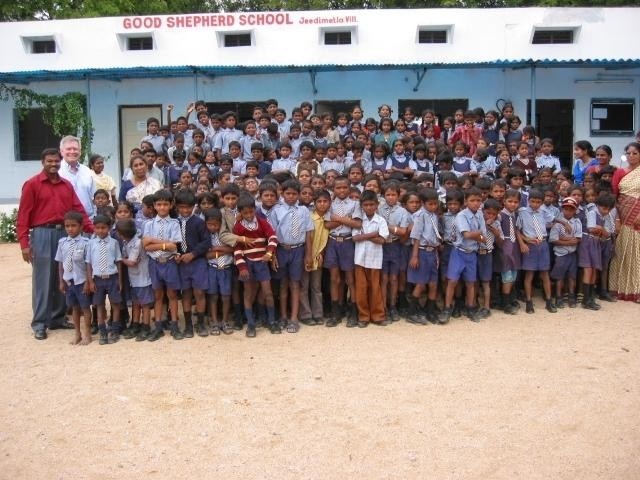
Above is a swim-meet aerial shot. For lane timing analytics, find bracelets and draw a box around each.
[266,252,272,258]
[394,225,397,235]
[215,250,219,259]
[162,242,165,251]
[242,235,246,243]
[615,219,620,221]
[479,234,484,242]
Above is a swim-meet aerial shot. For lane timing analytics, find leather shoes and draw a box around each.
[233,317,244,329]
[526,300,535,314]
[35,328,46,339]
[246,323,256,336]
[277,319,286,328]
[150,330,163,340]
[194,321,208,338]
[90,322,99,335]
[136,328,149,341]
[580,299,601,310]
[99,332,106,345]
[267,321,281,332]
[391,298,522,324]
[110,326,122,342]
[170,321,183,339]
[125,328,137,339]
[601,291,614,301]
[51,322,74,330]
[558,299,565,308]
[547,301,555,311]
[569,297,577,306]
[185,321,194,337]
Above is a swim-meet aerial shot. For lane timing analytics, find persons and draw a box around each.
[335,141,346,164]
[121,147,141,181]
[454,109,465,128]
[191,146,204,160]
[402,107,418,138]
[472,146,490,172]
[335,113,350,144]
[154,152,171,188]
[228,141,247,177]
[553,169,574,193]
[440,187,463,318]
[474,108,485,129]
[323,168,339,200]
[412,144,434,183]
[370,167,384,188]
[412,133,427,146]
[506,116,523,142]
[187,123,196,131]
[111,200,136,333]
[140,117,165,155]
[499,121,509,139]
[313,143,330,175]
[203,208,235,335]
[598,168,614,183]
[460,174,475,196]
[489,179,507,211]
[414,170,434,192]
[244,160,263,186]
[496,162,513,191]
[101,206,117,234]
[274,109,291,144]
[298,170,311,187]
[349,121,362,140]
[178,117,195,148]
[548,197,583,308]
[399,191,423,319]
[583,187,597,204]
[512,141,537,187]
[85,214,123,345]
[170,121,179,145]
[343,133,350,158]
[386,140,414,184]
[609,142,640,304]
[272,168,295,187]
[321,112,339,146]
[192,193,218,221]
[535,138,561,175]
[264,145,277,166]
[437,171,458,210]
[167,104,174,125]
[127,149,166,188]
[16,148,95,341]
[256,122,281,151]
[140,195,157,238]
[173,189,211,338]
[363,175,386,213]
[295,141,321,176]
[497,190,523,314]
[472,177,491,205]
[287,124,303,158]
[437,184,487,325]
[348,105,365,127]
[323,175,362,328]
[364,136,374,152]
[533,165,557,191]
[473,137,493,165]
[595,182,618,303]
[540,189,560,299]
[377,105,392,119]
[578,193,615,310]
[232,196,278,338]
[423,125,436,144]
[204,151,223,179]
[250,142,272,178]
[310,114,321,138]
[434,152,462,190]
[450,110,483,157]
[140,141,153,153]
[452,140,477,178]
[311,124,331,150]
[352,190,390,328]
[189,128,211,156]
[440,116,455,145]
[347,131,371,161]
[500,102,514,123]
[377,179,407,323]
[187,153,203,183]
[348,186,362,203]
[477,199,504,321]
[58,135,96,224]
[271,141,295,176]
[297,182,314,212]
[216,170,231,188]
[344,136,356,158]
[197,112,215,142]
[585,144,618,194]
[185,100,208,128]
[557,180,574,201]
[399,181,417,206]
[232,177,245,193]
[265,99,278,124]
[196,164,213,191]
[214,111,242,160]
[288,102,312,122]
[484,110,505,154]
[257,115,271,134]
[218,154,238,183]
[157,125,171,149]
[493,149,512,170]
[402,137,414,159]
[116,219,154,342]
[369,142,387,179]
[88,154,119,211]
[256,183,277,232]
[166,133,189,165]
[407,188,444,326]
[91,190,116,224]
[267,180,313,333]
[253,107,264,132]
[300,120,314,144]
[54,209,92,346]
[298,189,329,325]
[219,180,256,331]
[573,140,599,188]
[259,180,281,204]
[321,144,345,175]
[345,142,369,175]
[174,169,194,194]
[347,164,365,195]
[142,189,184,342]
[418,109,440,140]
[240,120,256,161]
[394,119,406,140]
[292,107,303,131]
[310,175,325,194]
[581,172,598,192]
[198,176,210,190]
[523,125,540,156]
[375,119,398,149]
[210,187,224,207]
[195,183,209,195]
[363,118,380,136]
[244,177,261,202]
[569,185,585,232]
[494,140,508,157]
[425,141,438,168]
[165,148,190,190]
[517,188,572,314]
[507,141,520,161]
[207,112,225,150]
[504,166,529,209]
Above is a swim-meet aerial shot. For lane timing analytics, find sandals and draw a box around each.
[222,321,232,334]
[288,321,299,332]
[210,323,219,336]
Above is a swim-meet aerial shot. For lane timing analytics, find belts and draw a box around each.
[328,235,352,242]
[419,247,437,251]
[36,223,65,229]
[93,274,118,280]
[209,264,231,270]
[152,257,177,263]
[280,244,305,249]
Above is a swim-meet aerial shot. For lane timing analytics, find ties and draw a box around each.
[153,220,165,258]
[214,232,224,268]
[432,216,442,241]
[509,216,516,242]
[181,218,187,253]
[67,240,75,271]
[486,229,494,250]
[561,226,570,241]
[450,216,457,242]
[99,240,108,271]
[532,211,542,241]
[385,207,390,226]
[336,199,344,230]
[292,208,298,239]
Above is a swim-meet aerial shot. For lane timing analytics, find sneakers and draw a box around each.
[326,317,342,328]
[316,318,324,325]
[302,318,314,326]
[346,317,356,327]
[375,321,388,326]
[357,322,368,327]
